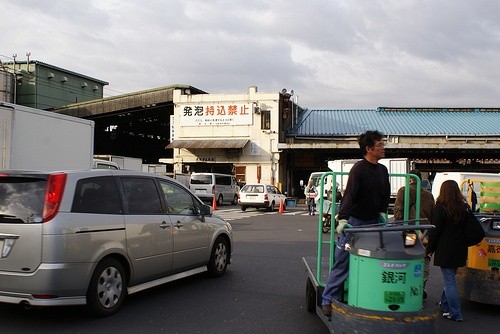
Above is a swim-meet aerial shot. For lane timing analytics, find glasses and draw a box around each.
[370,144,385,149]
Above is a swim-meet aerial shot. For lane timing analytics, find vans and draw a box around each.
[189,172,240,205]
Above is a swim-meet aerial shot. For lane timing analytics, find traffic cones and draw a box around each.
[210,197,218,210]
[278,198,285,213]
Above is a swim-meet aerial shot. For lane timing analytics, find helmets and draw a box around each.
[330,181,339,189]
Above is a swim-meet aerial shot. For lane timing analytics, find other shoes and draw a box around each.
[309,212,315,216]
[322,304,331,316]
[443,312,455,319]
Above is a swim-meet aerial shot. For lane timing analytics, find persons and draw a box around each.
[321,130,391,315]
[304,180,318,216]
[426,180,486,319]
[392,170,436,301]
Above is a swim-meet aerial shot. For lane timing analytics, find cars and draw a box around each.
[237,184,287,212]
[0,169,235,319]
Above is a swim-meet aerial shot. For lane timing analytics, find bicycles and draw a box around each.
[322,212,337,232]
[307,193,316,216]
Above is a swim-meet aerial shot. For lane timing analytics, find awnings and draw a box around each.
[165,139,249,149]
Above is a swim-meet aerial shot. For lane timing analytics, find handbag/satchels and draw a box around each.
[464,204,485,247]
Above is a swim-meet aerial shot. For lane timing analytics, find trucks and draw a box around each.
[93,155,142,172]
[300,158,415,212]
[430,171,500,209]
[0,101,119,171]
[142,164,166,176]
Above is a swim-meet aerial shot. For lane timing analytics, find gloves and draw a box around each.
[336,219,352,237]
